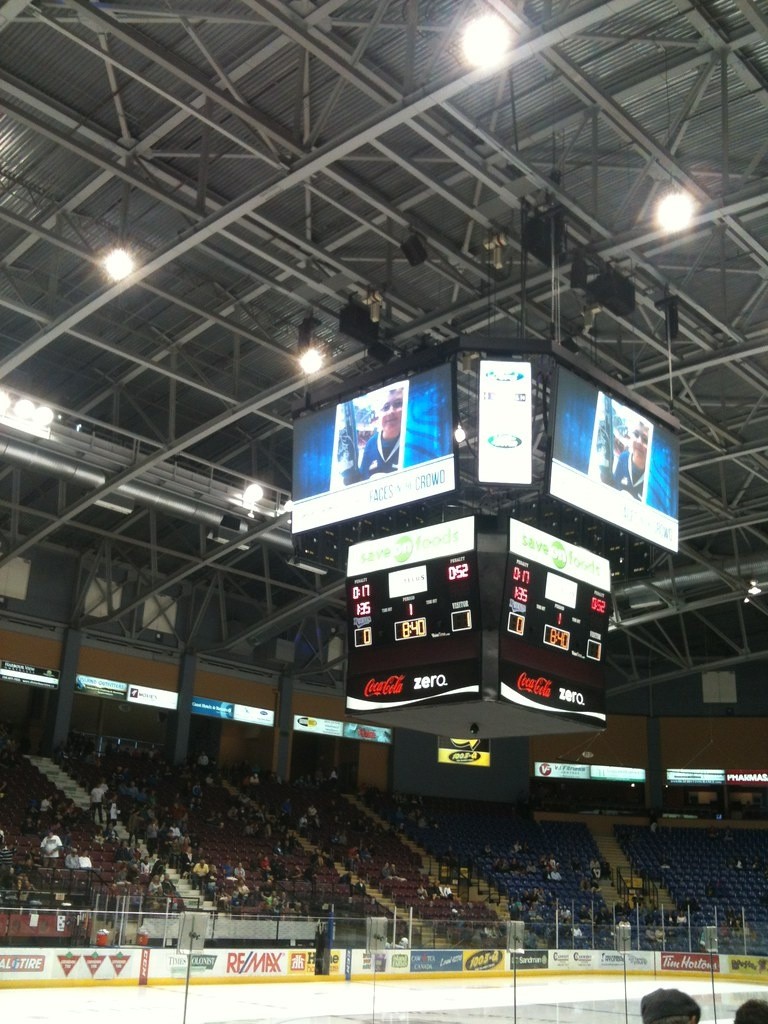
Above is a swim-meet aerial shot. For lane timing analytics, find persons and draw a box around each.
[734,999,768,1024]
[612,418,651,503]
[0,745,767,957]
[361,385,404,479]
[640,988,701,1024]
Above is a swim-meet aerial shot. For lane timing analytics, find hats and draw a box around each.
[641,988,700,1024]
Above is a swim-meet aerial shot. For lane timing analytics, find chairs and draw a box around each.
[0,749,768,955]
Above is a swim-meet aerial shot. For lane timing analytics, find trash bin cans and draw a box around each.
[97,929,110,946]
[136,926,149,946]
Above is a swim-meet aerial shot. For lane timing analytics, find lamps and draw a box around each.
[295,318,324,374]
[400,235,429,268]
